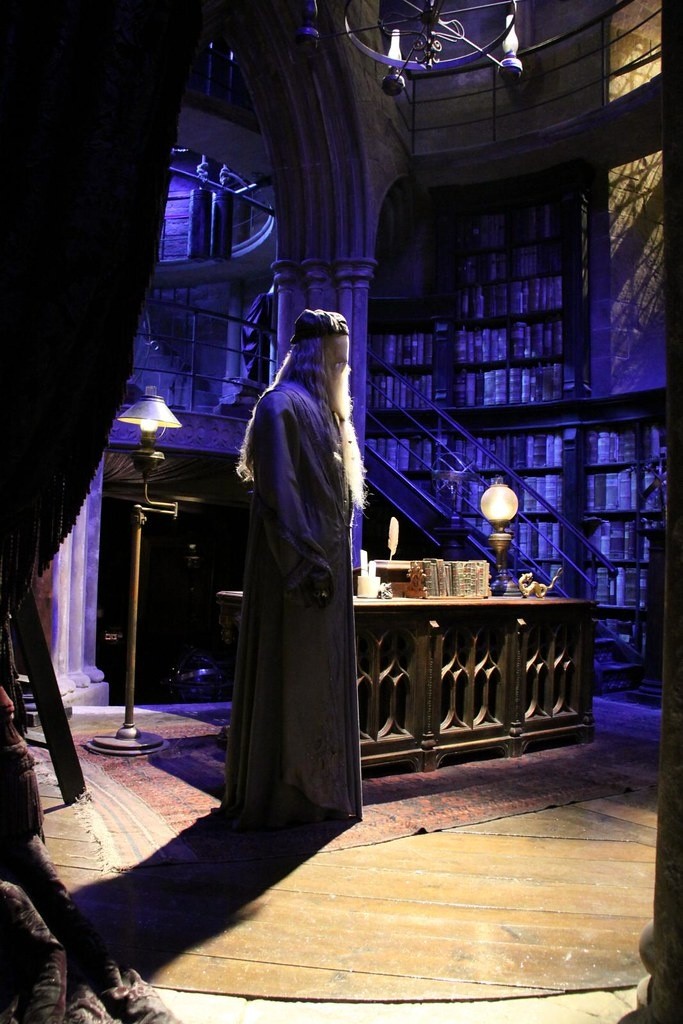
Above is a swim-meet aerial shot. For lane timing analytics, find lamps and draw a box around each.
[481,483,523,597]
[297,1,524,94]
[83,392,182,755]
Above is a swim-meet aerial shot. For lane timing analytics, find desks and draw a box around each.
[214,591,600,774]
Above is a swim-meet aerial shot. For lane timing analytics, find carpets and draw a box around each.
[26,696,669,872]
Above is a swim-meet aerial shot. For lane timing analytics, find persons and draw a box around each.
[212,310,368,829]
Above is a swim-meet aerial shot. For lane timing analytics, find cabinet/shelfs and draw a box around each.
[368,211,666,680]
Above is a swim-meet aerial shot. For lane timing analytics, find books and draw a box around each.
[534,562,648,653]
[365,198,668,558]
[411,558,490,597]
